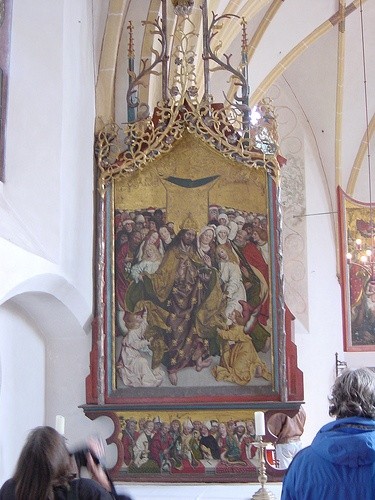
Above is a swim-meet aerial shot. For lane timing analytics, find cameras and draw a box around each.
[70,447,100,465]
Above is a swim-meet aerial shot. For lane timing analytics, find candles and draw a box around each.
[254,411,266,436]
[55,415,66,438]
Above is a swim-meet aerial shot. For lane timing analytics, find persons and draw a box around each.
[0,426,133,500]
[280,368,375,499]
